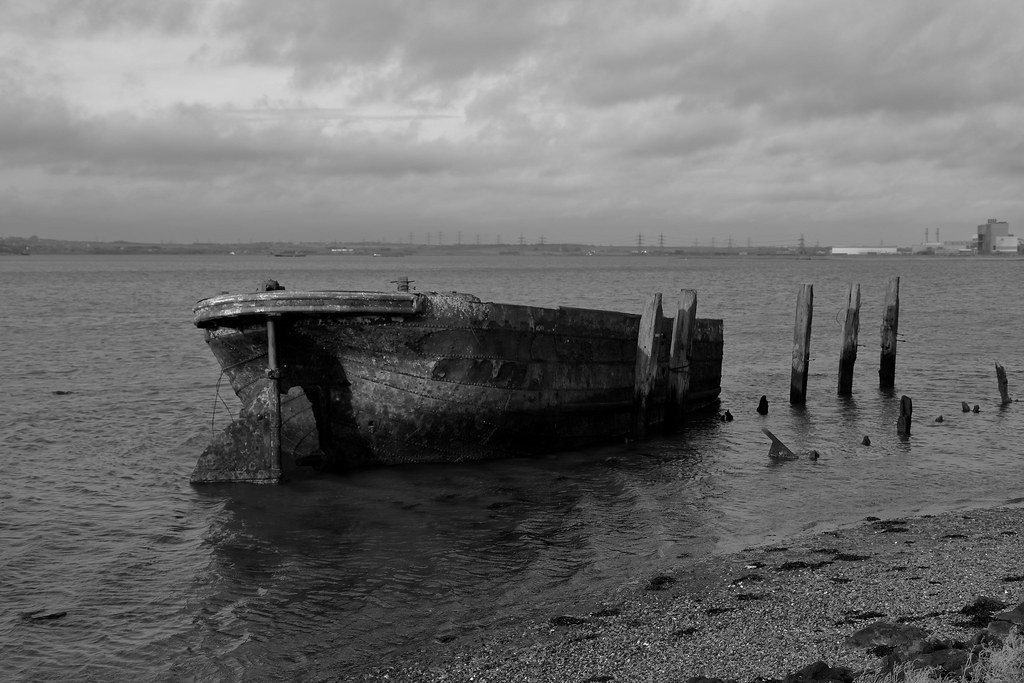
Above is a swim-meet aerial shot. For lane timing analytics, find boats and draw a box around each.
[189,275,724,489]
